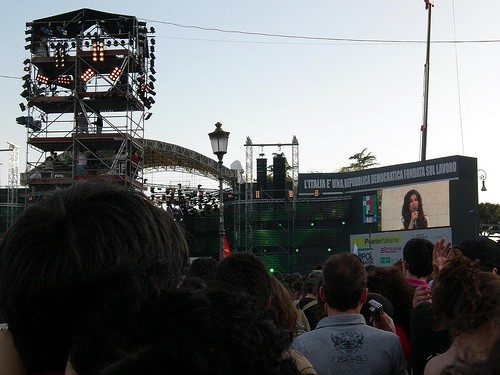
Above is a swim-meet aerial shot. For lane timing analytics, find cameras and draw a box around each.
[369,300,384,324]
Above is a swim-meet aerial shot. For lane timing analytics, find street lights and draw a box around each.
[208,121,230,262]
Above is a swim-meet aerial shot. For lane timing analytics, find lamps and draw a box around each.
[478,169,487,191]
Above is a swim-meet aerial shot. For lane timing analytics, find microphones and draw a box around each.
[414,208,418,227]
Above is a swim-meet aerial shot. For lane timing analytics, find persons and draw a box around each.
[50,149,58,162]
[63,238,500,375]
[95,111,103,133]
[77,149,87,178]
[86,149,98,176]
[132,151,139,179]
[0,177,189,375]
[400,190,428,230]
[78,110,88,133]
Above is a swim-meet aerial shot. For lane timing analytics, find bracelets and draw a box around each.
[300,366,314,373]
